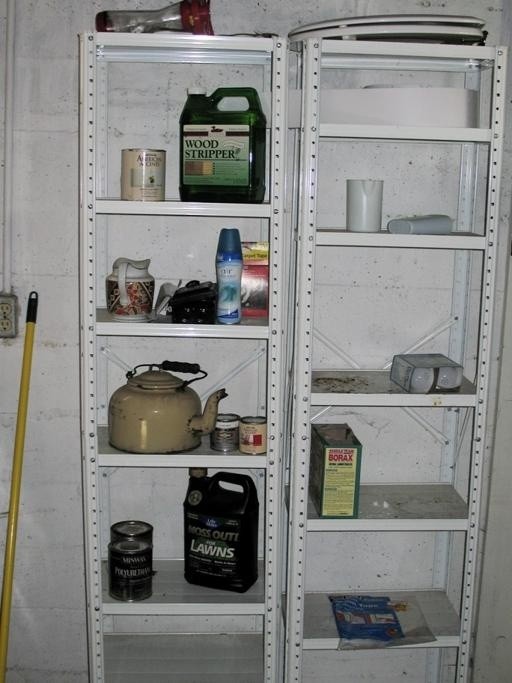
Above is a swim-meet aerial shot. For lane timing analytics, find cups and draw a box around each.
[345,179,384,232]
[212,412,268,455]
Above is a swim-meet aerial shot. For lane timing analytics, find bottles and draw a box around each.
[216,227,244,325]
[109,520,154,602]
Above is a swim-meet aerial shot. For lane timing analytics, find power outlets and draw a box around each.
[1,295,17,338]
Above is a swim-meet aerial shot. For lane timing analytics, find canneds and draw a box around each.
[121,147,166,201]
[110,520,154,543]
[107,543,154,603]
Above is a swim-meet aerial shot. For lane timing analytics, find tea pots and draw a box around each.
[107,360,228,454]
[105,256,155,322]
